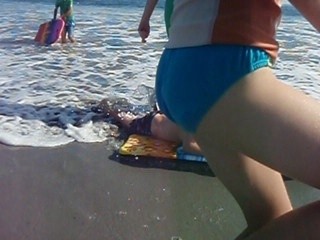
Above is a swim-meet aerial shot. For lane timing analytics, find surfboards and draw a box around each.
[34,19,65,45]
[119,134,209,163]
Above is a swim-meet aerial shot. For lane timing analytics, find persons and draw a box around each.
[138,0,320,240]
[54,0,76,43]
[96,100,204,156]
